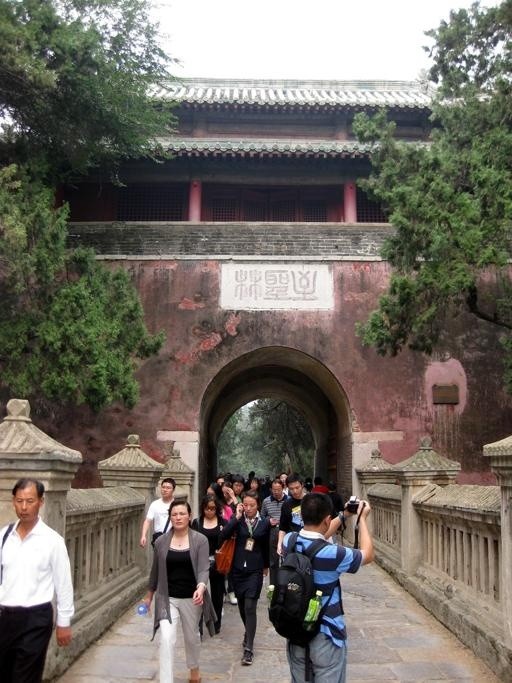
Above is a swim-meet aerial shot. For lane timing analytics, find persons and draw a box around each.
[218,492,271,665]
[140,478,176,615]
[282,493,375,683]
[143,500,218,683]
[192,471,344,644]
[0,478,75,683]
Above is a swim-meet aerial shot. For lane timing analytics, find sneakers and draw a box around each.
[215,627,220,633]
[241,632,255,665]
[226,591,238,604]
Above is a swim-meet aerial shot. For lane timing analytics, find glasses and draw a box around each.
[206,506,216,511]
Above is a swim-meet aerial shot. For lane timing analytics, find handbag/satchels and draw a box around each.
[151,532,163,548]
[214,531,237,575]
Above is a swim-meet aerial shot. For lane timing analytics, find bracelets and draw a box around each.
[338,511,347,522]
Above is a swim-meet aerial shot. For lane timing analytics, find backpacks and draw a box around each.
[268,531,339,648]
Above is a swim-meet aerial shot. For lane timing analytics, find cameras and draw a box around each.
[346,494,364,513]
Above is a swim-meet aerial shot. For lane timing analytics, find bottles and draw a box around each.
[137,605,151,621]
[304,589,324,621]
[266,585,276,610]
